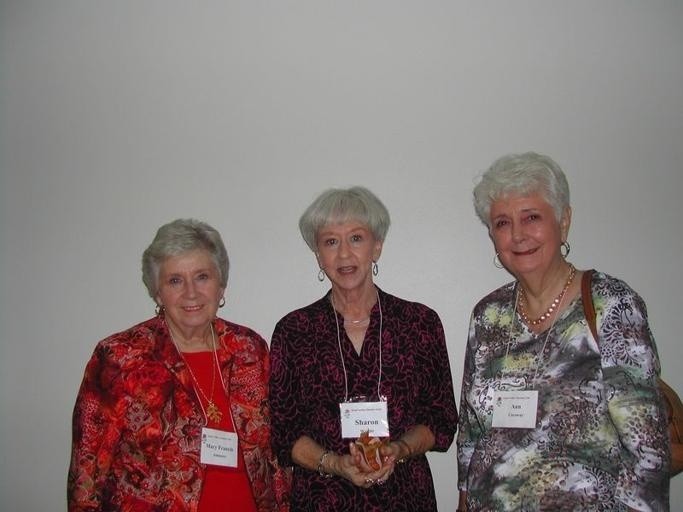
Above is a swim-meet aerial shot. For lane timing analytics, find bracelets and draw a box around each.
[316,453,332,480]
[391,443,408,466]
[399,438,412,457]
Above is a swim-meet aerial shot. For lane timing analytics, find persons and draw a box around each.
[451,151,672,512]
[265,185,458,512]
[67,214,293,512]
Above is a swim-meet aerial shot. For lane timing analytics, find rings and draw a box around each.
[366,477,375,486]
[384,454,394,463]
[378,479,384,487]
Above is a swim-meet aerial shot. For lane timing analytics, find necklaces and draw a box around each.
[166,326,223,425]
[343,317,371,326]
[515,265,574,325]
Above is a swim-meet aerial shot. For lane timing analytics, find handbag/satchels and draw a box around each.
[578,267,683,479]
